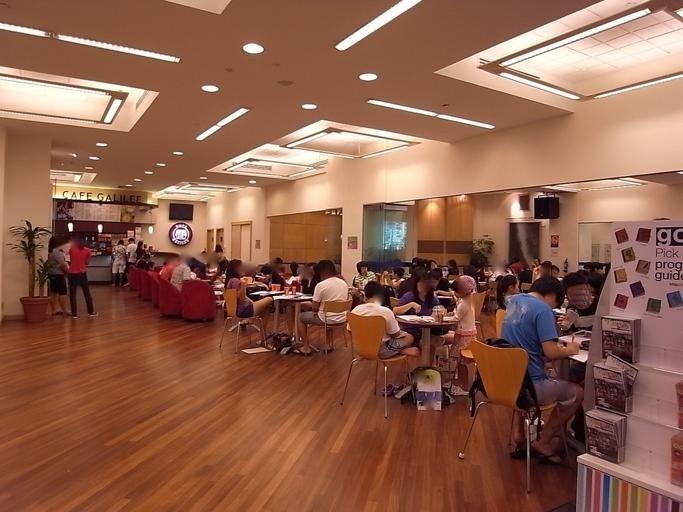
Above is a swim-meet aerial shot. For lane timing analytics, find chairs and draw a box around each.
[495,308,507,340]
[218,288,266,354]
[306,301,352,362]
[341,312,416,420]
[457,340,569,495]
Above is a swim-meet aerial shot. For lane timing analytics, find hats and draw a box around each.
[457,275,477,293]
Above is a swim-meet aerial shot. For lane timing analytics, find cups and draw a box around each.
[561,310,579,331]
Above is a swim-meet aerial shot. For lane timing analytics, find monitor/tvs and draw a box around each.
[169,203,194,220]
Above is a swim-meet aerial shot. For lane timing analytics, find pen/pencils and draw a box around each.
[571,335,575,343]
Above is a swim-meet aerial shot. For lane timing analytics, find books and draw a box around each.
[600,314,641,364]
[592,354,638,410]
[584,405,627,464]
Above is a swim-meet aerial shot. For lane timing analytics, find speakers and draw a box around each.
[533,196,559,219]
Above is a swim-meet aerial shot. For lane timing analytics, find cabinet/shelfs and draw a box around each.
[575,221,682,512]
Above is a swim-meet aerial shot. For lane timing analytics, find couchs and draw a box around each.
[157,277,181,318]
[181,279,214,322]
[135,269,140,295]
[151,274,159,308]
[140,270,152,303]
[128,265,135,292]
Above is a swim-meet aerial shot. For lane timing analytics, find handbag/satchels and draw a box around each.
[272,332,292,352]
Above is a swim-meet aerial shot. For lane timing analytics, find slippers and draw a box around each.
[381,383,398,395]
[510,445,531,458]
[448,386,469,396]
[530,450,562,465]
[393,383,407,395]
[443,378,455,389]
[292,348,312,355]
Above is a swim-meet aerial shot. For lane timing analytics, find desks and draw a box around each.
[253,289,314,355]
[557,330,590,439]
[394,314,460,405]
[76,247,127,285]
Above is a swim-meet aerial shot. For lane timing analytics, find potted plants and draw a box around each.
[5,219,58,322]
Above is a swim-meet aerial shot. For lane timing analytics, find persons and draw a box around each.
[261,256,286,285]
[500,275,584,463]
[192,263,218,282]
[529,261,560,291]
[583,264,591,272]
[48,237,69,316]
[136,240,146,256]
[446,275,477,395]
[67,232,98,318]
[393,271,440,371]
[137,254,150,269]
[287,262,302,293]
[380,271,388,286]
[301,262,321,295]
[591,262,604,275]
[497,275,518,309]
[352,261,376,298]
[398,266,425,298]
[392,268,406,288]
[123,238,137,287]
[511,257,524,274]
[448,259,459,276]
[532,259,540,268]
[556,273,598,335]
[111,240,127,286]
[346,281,420,357]
[433,268,450,296]
[447,265,489,311]
[224,259,273,345]
[292,259,348,356]
[425,259,437,272]
[170,264,190,290]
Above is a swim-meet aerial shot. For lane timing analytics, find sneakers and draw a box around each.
[72,314,80,319]
[88,311,98,317]
